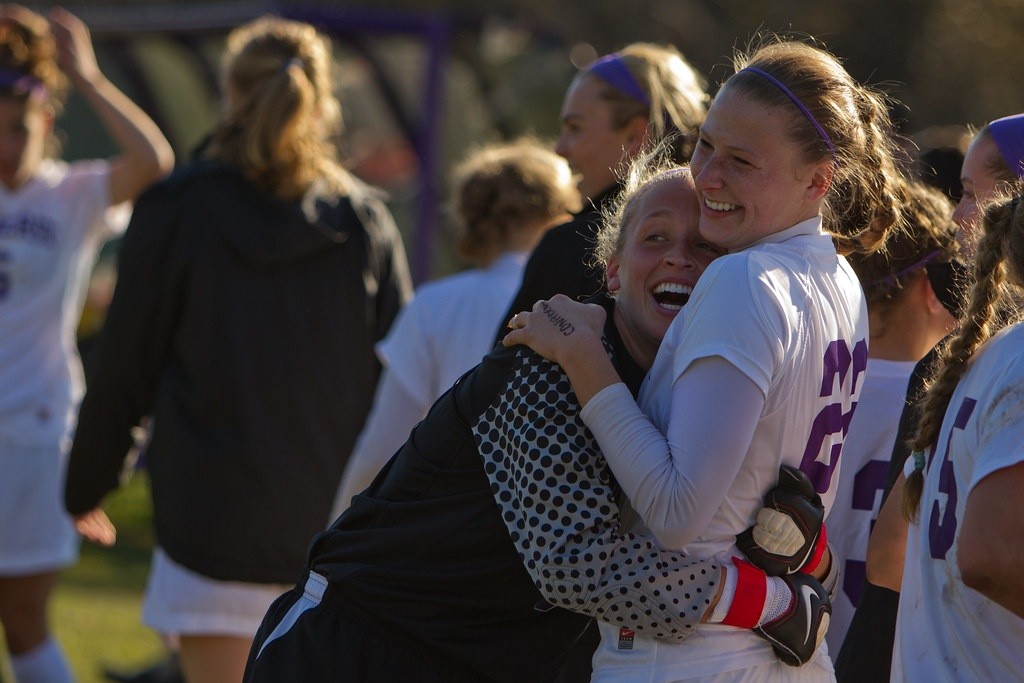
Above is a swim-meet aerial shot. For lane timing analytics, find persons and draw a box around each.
[0,6,173,682]
[494,40,707,344]
[503,40,894,683]
[243,167,836,683]
[65,14,415,683]
[828,113,1024,664]
[326,139,581,527]
[835,197,1024,683]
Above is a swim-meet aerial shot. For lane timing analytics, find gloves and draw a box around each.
[754,555,832,668]
[735,464,826,576]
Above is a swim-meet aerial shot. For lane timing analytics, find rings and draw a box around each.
[513,315,519,329]
